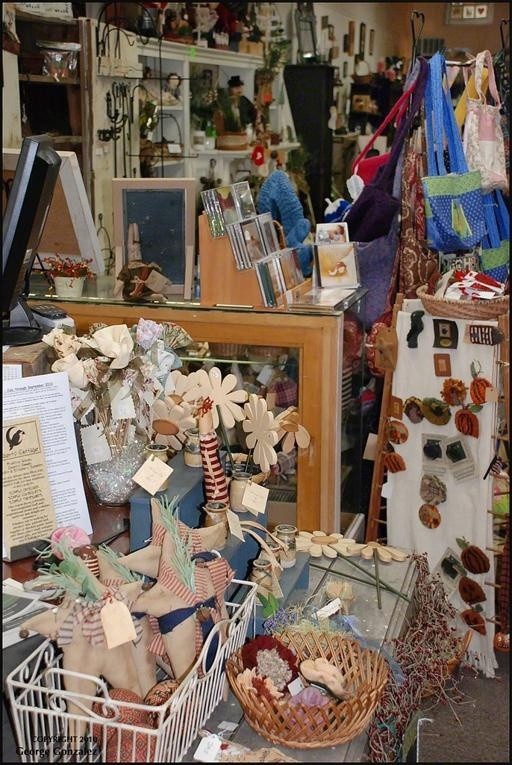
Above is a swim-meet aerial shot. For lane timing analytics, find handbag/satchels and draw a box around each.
[328,53,421,322]
[422,50,510,281]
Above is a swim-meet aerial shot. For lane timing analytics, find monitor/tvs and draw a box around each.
[2,135,61,345]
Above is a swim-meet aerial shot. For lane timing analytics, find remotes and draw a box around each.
[29,303,67,319]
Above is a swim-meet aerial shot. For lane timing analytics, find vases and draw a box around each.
[54,277,86,297]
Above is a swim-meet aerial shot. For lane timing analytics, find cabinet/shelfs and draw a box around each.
[284,62,337,224]
[27,287,369,534]
[15,14,144,278]
[138,36,301,209]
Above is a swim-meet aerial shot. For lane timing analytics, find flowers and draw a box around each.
[41,318,193,454]
[34,252,94,281]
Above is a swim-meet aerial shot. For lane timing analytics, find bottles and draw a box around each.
[205,501,229,542]
[251,559,271,607]
[184,427,205,469]
[276,524,297,569]
[145,444,168,493]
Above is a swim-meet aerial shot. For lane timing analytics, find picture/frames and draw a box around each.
[112,178,195,299]
[3,148,105,274]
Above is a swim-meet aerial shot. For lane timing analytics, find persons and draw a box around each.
[226,74,255,130]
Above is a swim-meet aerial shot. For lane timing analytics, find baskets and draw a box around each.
[224,628,392,752]
[415,277,509,322]
[6,575,259,761]
